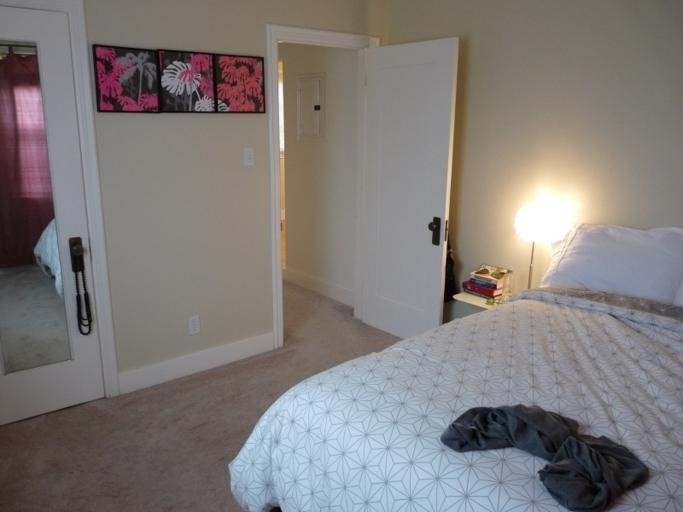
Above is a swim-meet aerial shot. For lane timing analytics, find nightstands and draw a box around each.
[450,294,511,322]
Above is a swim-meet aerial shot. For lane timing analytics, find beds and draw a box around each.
[227,253,683,511]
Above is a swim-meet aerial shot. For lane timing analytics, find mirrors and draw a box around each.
[0,39,73,376]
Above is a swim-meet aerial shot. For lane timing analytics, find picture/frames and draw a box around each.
[91,42,160,113]
[156,46,217,114]
[213,52,265,114]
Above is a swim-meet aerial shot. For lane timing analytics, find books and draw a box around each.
[461,264,514,300]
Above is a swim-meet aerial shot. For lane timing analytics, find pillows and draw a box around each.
[539,222,683,305]
